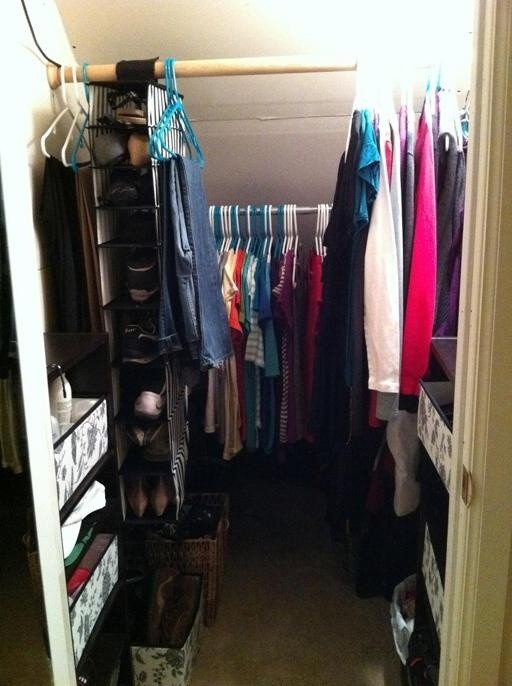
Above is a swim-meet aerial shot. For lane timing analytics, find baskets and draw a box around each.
[124,490,231,628]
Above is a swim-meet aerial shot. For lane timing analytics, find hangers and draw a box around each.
[148,58,206,169]
[42,63,90,172]
[344,54,472,164]
[206,203,330,290]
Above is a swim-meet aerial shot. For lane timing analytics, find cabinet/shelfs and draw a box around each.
[44,331,132,686]
[405,337,458,686]
[87,79,189,523]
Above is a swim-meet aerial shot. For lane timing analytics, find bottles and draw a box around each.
[50,363,71,425]
[50,416,60,442]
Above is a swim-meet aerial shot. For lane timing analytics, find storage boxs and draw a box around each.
[421,519,449,648]
[51,391,110,514]
[415,378,456,492]
[130,572,206,686]
[65,531,120,670]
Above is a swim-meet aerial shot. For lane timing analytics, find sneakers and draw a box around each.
[162,573,200,648]
[145,565,179,646]
[93,90,175,518]
